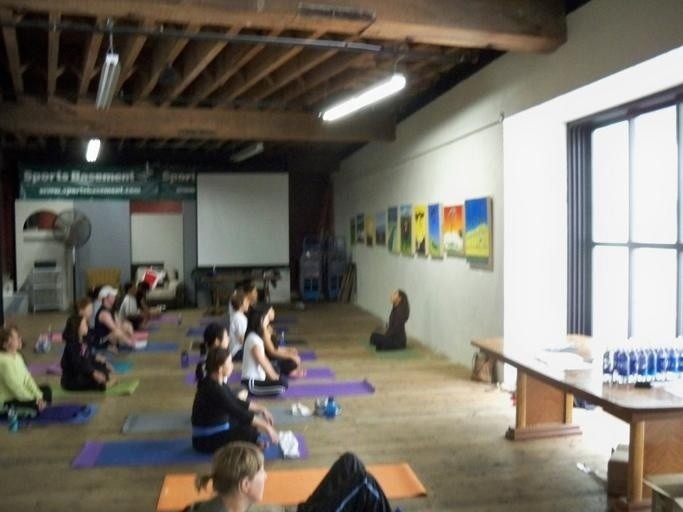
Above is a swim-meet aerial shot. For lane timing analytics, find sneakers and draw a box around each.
[32,331,62,353]
[290,398,343,420]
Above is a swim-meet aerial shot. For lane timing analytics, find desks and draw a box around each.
[472,333,683,511]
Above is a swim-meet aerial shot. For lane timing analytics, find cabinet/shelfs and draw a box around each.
[32,263,64,314]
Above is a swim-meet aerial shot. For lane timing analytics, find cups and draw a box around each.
[176,312,184,327]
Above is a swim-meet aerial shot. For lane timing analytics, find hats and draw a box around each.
[98,286,118,299]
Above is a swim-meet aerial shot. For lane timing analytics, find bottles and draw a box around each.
[35,331,46,352]
[279,330,287,347]
[7,405,19,431]
[180,351,189,368]
[326,396,338,419]
[603,346,683,384]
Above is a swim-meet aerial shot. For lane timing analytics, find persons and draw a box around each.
[370,290,409,351]
[60,281,161,391]
[191,280,392,511]
[0,326,52,421]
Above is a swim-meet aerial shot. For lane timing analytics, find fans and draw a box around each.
[53,209,92,319]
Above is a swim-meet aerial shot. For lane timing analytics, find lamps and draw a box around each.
[94,18,123,112]
[317,52,407,123]
